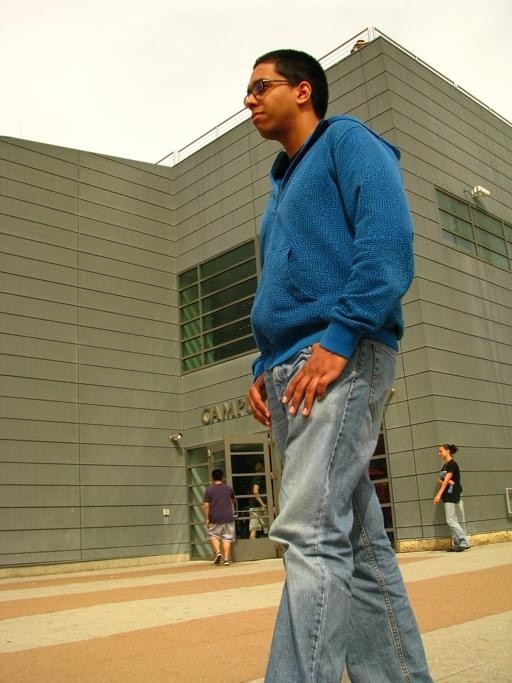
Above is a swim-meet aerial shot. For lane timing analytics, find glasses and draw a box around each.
[244,79,314,108]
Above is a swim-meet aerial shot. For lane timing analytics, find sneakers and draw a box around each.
[223,561,229,565]
[215,552,222,564]
[448,547,471,551]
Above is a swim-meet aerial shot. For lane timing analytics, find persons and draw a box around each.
[245,48,434,683]
[247,462,270,539]
[203,469,238,565]
[432,444,471,552]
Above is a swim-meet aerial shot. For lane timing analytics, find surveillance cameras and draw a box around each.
[471,185,491,199]
